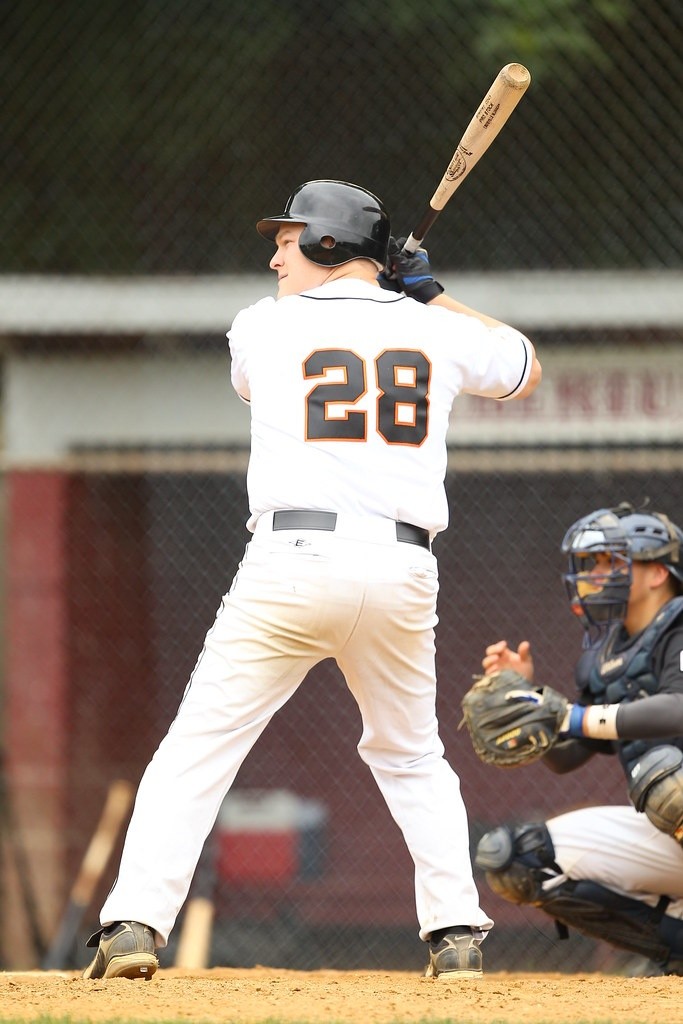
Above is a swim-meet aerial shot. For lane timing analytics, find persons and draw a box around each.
[475,503,683,976]
[84,181,543,981]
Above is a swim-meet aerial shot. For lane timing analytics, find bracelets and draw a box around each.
[569,703,585,738]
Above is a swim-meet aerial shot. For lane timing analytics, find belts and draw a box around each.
[273,510,430,552]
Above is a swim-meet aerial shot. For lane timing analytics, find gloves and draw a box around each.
[376,236,445,304]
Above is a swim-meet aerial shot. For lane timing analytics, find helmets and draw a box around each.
[562,513,683,563]
[257,180,391,275]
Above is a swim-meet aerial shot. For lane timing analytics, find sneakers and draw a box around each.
[82,922,159,981]
[426,934,483,979]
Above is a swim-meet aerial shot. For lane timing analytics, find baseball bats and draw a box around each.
[391,63,531,274]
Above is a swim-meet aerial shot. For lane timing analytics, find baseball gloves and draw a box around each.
[455,669,568,770]
